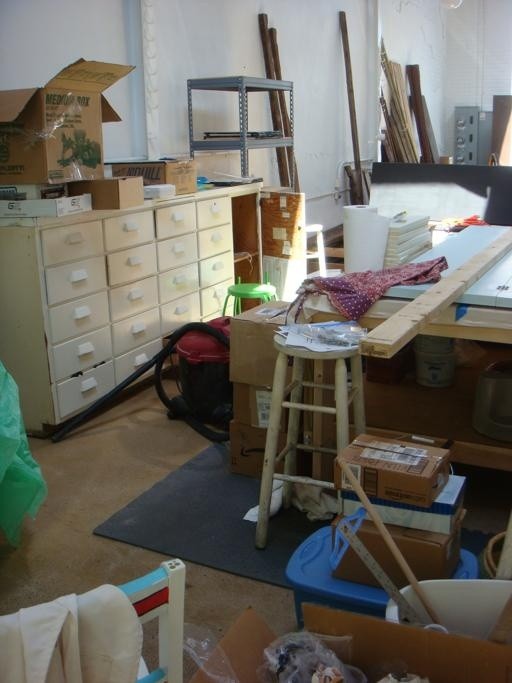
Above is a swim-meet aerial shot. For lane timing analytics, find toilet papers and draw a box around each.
[343,205,390,274]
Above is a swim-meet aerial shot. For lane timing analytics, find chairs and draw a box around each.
[0,557,186,683]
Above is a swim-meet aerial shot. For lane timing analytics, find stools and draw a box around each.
[222,284,278,316]
[307,223,328,274]
[255,324,366,548]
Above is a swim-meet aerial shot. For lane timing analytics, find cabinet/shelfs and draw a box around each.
[187,76,296,191]
[0,181,269,441]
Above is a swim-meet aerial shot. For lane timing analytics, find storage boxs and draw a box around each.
[112,160,198,194]
[0,59,137,187]
[68,175,146,210]
[236,383,287,433]
[330,515,464,591]
[189,600,512,683]
[337,476,468,536]
[287,520,480,632]
[333,436,455,509]
[229,300,309,389]
[227,420,288,476]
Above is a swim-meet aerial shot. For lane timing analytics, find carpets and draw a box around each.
[90,439,330,590]
